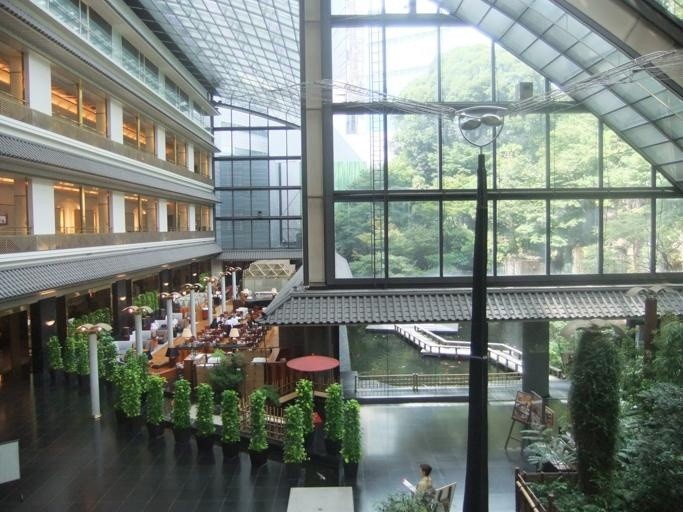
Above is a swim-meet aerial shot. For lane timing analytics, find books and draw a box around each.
[400,479,416,494]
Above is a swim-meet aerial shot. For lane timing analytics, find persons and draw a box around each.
[212,311,241,328]
[412,464,432,497]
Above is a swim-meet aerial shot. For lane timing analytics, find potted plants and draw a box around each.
[45,335,123,402]
[144,377,165,436]
[245,385,270,466]
[281,406,309,479]
[342,400,363,478]
[193,382,218,451]
[322,382,345,456]
[171,378,192,443]
[219,389,241,456]
[116,348,150,418]
[294,379,314,450]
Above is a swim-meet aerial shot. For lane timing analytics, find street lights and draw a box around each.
[219,267,243,314]
[122,306,154,355]
[77,322,113,420]
[203,276,218,325]
[159,291,180,356]
[182,283,203,337]
